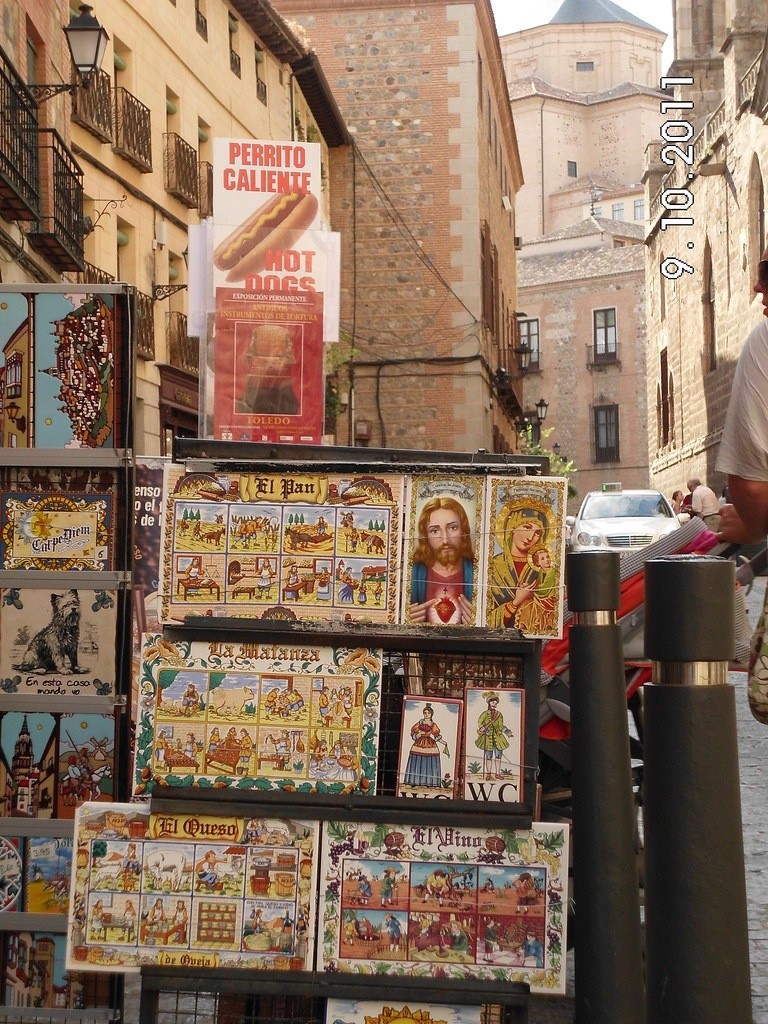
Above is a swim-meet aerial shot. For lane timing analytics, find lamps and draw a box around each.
[515,397,549,433]
[552,442,561,455]
[11,4,110,132]
[151,245,188,301]
[490,339,534,389]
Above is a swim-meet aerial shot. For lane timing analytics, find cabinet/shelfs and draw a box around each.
[139,437,550,1024]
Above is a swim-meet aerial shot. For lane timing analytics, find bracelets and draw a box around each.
[689,509,692,512]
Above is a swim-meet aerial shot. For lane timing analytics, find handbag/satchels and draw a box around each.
[747,582,768,725]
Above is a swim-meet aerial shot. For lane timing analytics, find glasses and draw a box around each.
[757,260,768,286]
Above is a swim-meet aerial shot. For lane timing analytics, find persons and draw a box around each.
[681,492,697,519]
[671,490,684,515]
[682,478,721,533]
[718,486,729,507]
[716,244,768,548]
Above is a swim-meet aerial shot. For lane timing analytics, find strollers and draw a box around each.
[497,517,768,1024]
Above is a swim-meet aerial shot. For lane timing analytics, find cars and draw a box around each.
[565,482,692,557]
